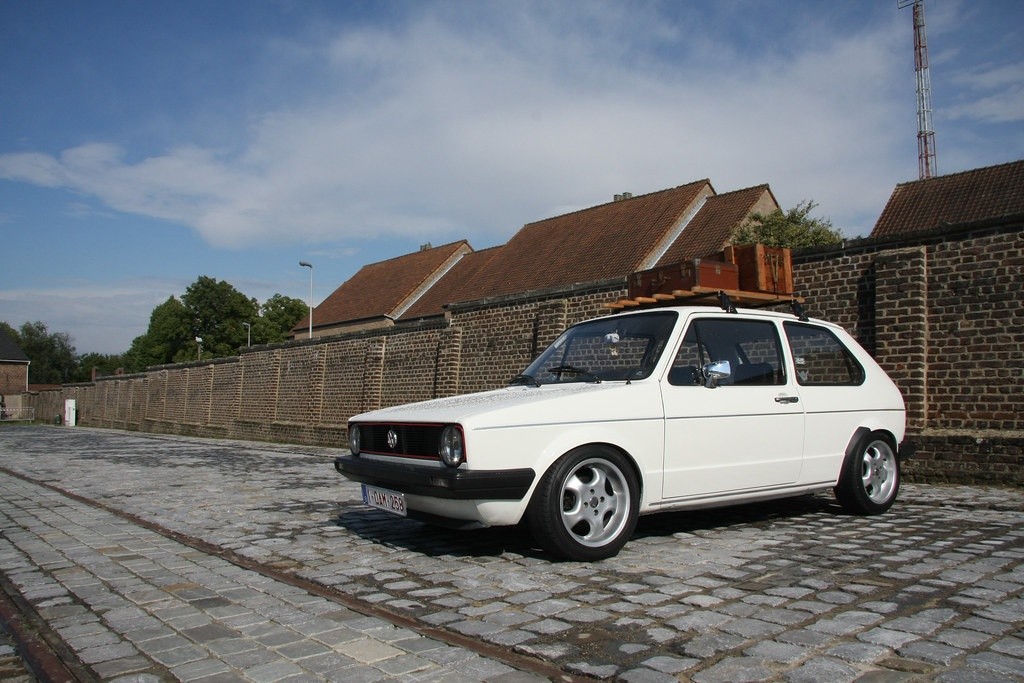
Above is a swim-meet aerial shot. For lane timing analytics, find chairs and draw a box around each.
[734,362,774,385]
[670,365,696,385]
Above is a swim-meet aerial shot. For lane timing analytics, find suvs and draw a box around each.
[334,292,908,563]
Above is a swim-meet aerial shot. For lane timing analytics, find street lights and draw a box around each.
[299,260,313,339]
[242,322,250,347]
